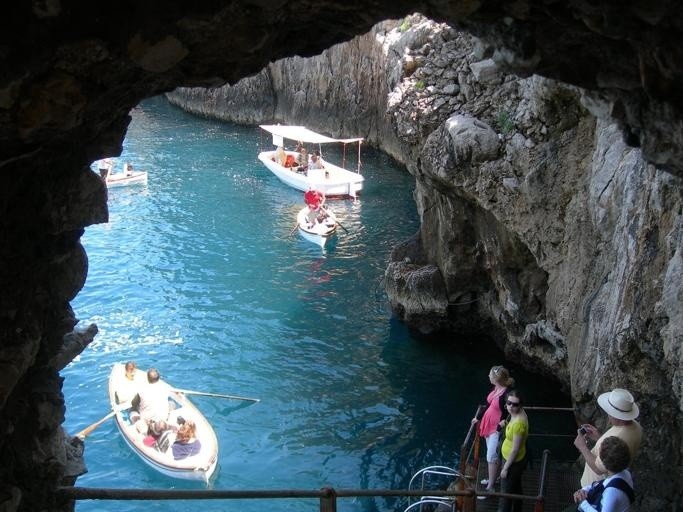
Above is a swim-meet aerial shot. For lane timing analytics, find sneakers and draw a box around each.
[477,487,495,500]
[481,476,501,485]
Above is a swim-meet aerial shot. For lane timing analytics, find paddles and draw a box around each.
[320,206,348,233]
[292,210,310,235]
[173,389,261,402]
[74,408,118,436]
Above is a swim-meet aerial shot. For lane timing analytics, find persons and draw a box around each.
[115,360,139,403]
[129,367,178,432]
[470,363,515,501]
[304,184,327,231]
[146,420,175,455]
[494,389,530,512]
[170,422,201,462]
[271,143,325,177]
[571,436,639,512]
[571,386,644,492]
[97,157,113,181]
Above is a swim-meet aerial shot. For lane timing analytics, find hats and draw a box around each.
[597,388,640,421]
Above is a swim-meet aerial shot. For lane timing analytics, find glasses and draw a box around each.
[506,400,521,408]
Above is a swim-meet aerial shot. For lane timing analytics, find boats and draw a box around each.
[109,363,219,483]
[297,206,337,247]
[258,125,365,197]
[106,171,148,189]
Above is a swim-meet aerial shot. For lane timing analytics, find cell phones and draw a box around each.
[580,427,588,435]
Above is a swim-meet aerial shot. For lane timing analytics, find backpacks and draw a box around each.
[577,477,636,512]
